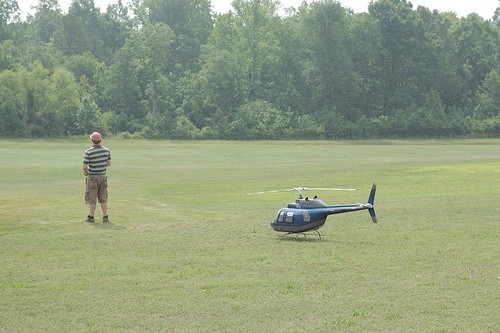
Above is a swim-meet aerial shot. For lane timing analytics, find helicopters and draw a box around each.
[246,182,378,243]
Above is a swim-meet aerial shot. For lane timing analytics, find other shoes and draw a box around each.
[103,215,109,222]
[84,215,95,222]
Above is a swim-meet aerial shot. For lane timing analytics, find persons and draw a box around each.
[82,131,111,221]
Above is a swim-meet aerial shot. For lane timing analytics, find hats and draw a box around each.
[90,132,102,140]
